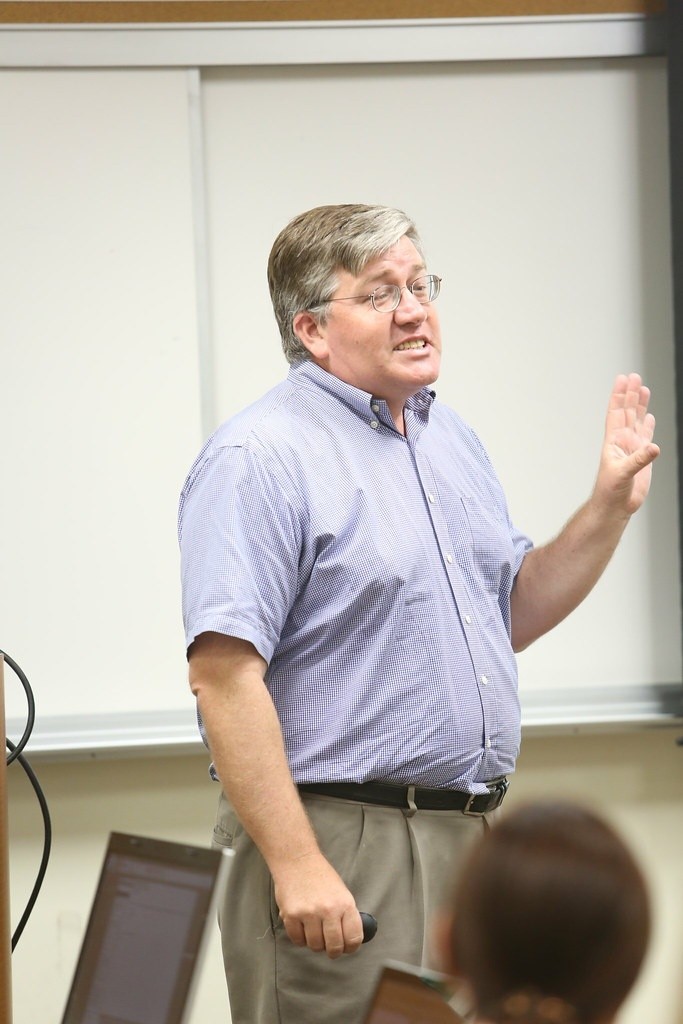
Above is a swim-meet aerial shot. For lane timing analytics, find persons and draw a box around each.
[178,204,660,1024]
[435,795,653,1024]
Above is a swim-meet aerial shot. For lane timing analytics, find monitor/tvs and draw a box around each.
[356,961,471,1024]
[58,829,236,1024]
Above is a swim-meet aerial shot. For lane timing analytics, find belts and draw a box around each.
[295,779,510,816]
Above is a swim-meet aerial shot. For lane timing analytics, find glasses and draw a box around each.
[313,274,443,313]
